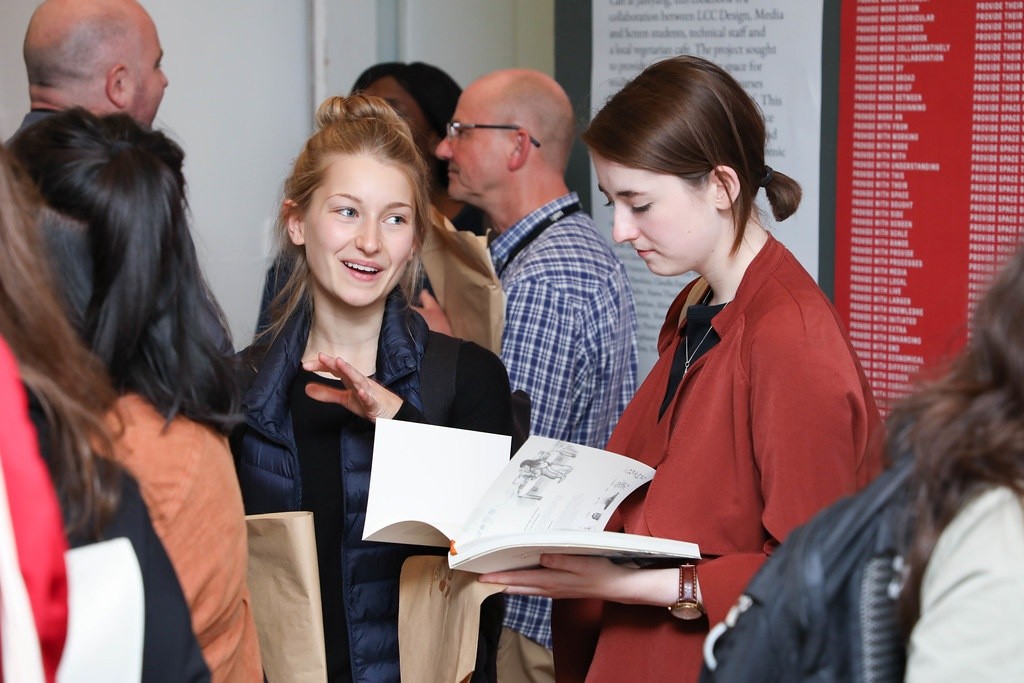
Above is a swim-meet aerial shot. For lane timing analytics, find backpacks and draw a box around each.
[699,449,1006,683]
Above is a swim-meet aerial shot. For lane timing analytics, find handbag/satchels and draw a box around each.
[240,511,328,683]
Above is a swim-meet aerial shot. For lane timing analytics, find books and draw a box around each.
[360,417,703,582]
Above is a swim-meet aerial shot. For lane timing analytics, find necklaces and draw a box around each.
[683,289,732,379]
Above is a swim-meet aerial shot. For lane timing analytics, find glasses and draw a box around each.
[446,121,541,148]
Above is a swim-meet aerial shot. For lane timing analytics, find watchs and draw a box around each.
[669,564,705,620]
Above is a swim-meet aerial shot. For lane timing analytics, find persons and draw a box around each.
[877,246,1024,683]
[477,55,879,683]
[229,92,515,683]
[253,61,483,360]
[0,106,272,683]
[17,0,168,137]
[404,69,640,683]
